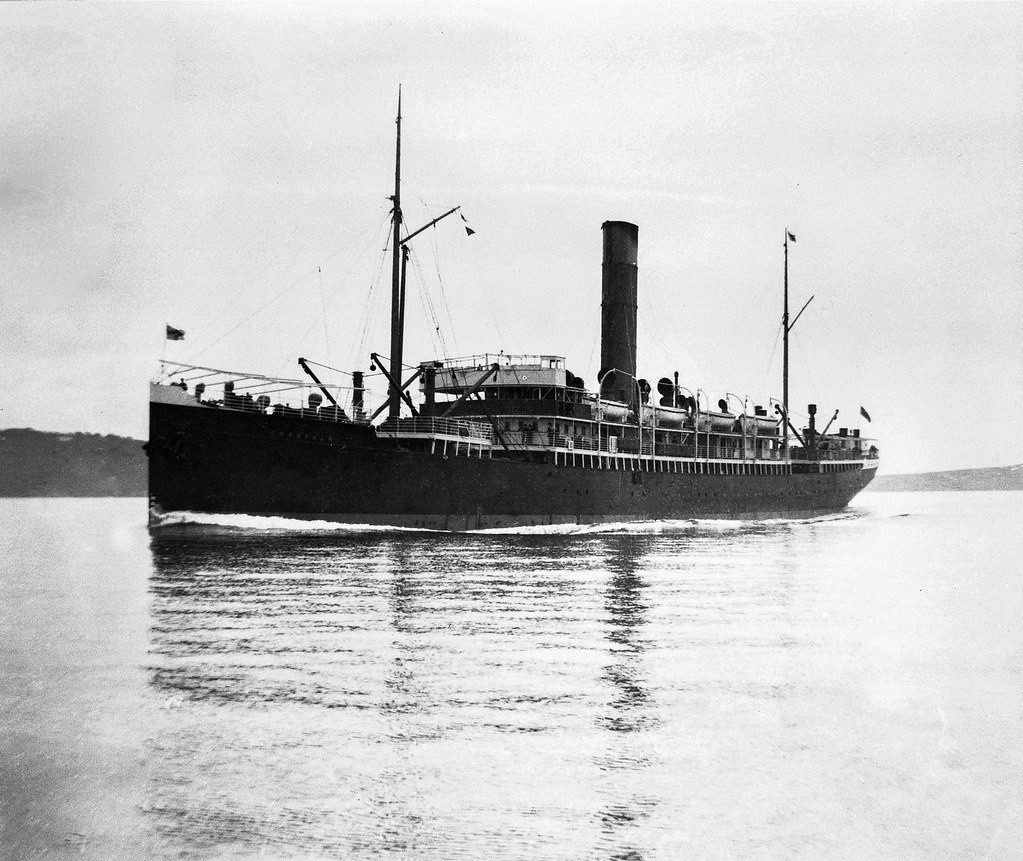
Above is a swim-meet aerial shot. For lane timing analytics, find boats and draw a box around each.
[142,83,880,545]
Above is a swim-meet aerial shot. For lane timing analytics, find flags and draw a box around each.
[789,233,796,241]
[861,406,871,422]
[166,325,185,340]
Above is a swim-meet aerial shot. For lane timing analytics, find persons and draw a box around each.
[180,378,188,391]
